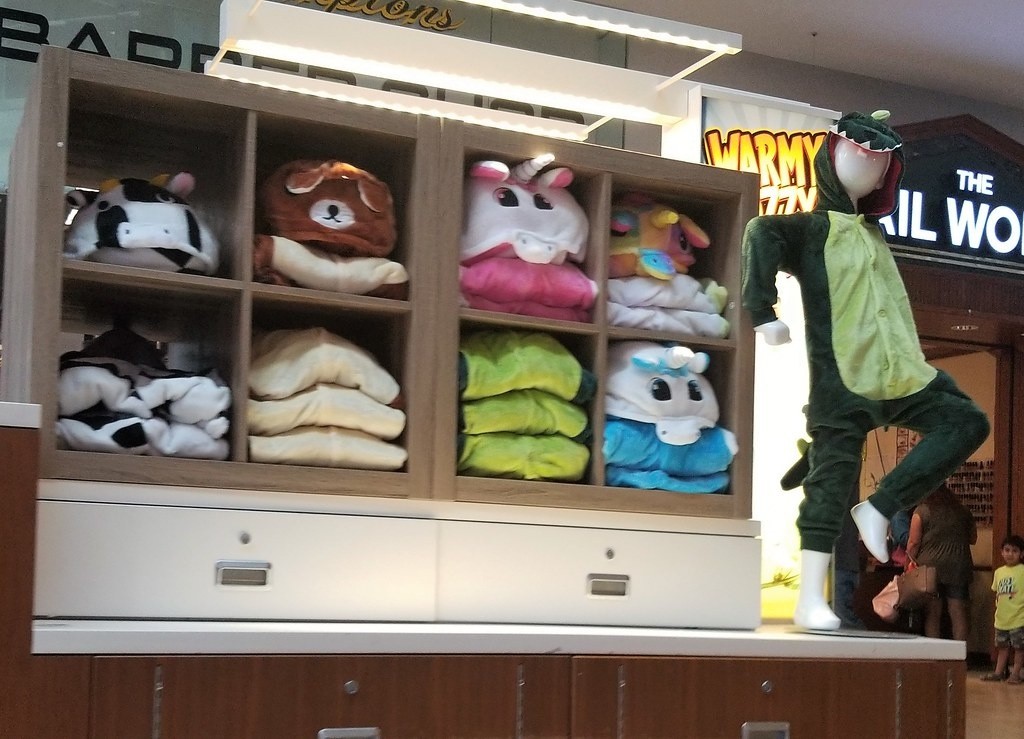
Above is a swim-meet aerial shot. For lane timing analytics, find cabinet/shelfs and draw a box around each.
[87,651,967,739]
[1,43,762,630]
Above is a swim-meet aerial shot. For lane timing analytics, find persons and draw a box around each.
[741,113,992,630]
[893,482,977,640]
[980,535,1024,684]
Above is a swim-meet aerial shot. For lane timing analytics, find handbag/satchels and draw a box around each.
[893,553,938,613]
[871,575,899,623]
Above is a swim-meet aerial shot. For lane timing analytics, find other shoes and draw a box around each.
[1006,675,1024,683]
[979,669,1010,681]
